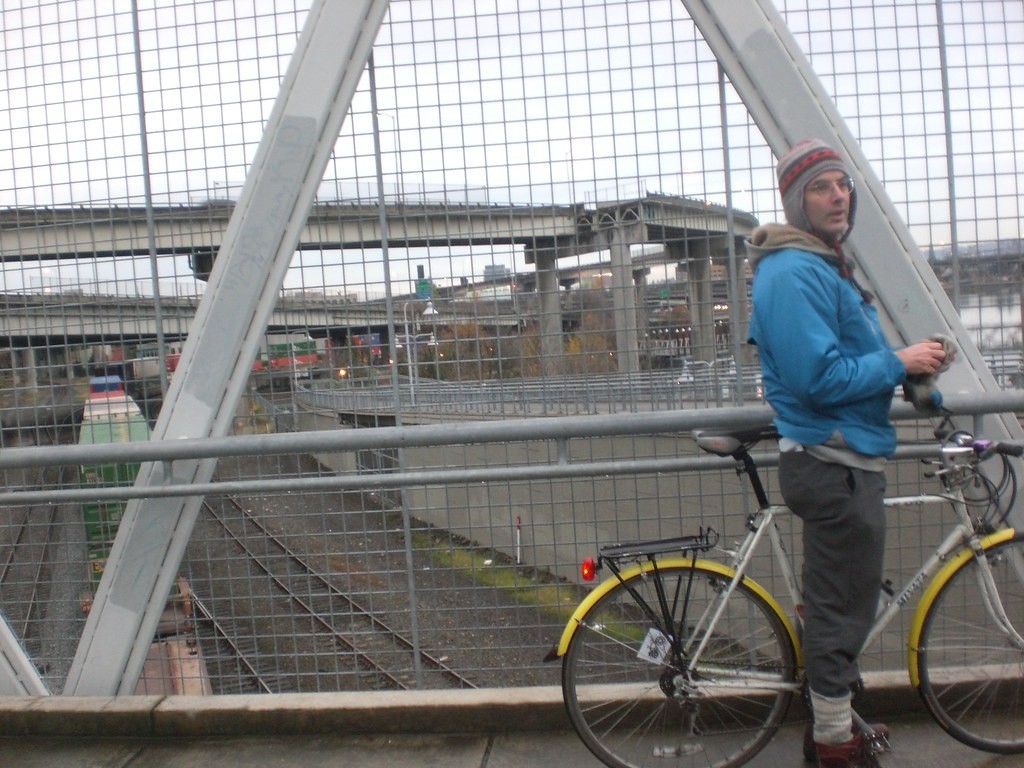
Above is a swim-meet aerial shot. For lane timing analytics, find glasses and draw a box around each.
[804,176,854,197]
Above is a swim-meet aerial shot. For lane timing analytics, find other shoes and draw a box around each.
[803,724,889,759]
[812,727,881,768]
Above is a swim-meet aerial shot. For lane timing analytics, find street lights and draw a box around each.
[290,331,314,394]
[676,355,737,398]
[403,293,437,407]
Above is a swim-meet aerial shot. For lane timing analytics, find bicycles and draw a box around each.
[542,424,1024,768]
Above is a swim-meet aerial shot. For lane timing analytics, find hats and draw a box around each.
[776,138,875,303]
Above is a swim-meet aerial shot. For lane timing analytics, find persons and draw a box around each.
[746,139,957,768]
